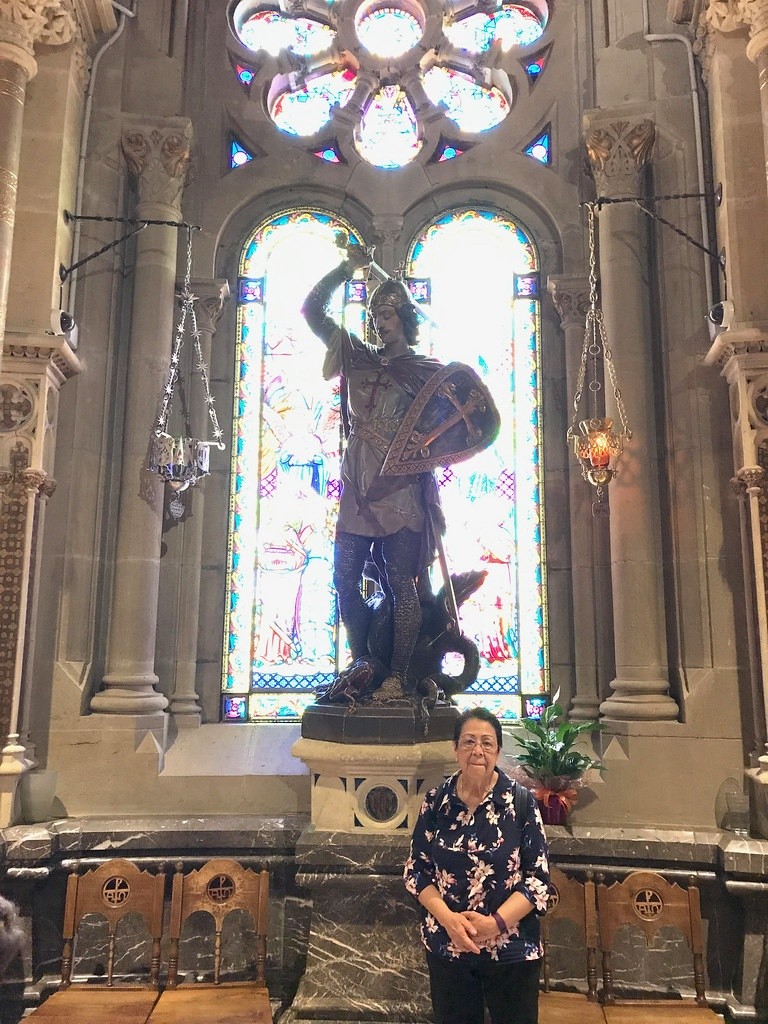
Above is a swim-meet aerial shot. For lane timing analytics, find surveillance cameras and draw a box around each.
[51,310,75,336]
[709,300,734,328]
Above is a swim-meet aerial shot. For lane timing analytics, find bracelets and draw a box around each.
[491,912,509,936]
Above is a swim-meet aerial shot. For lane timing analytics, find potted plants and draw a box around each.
[501,684,610,827]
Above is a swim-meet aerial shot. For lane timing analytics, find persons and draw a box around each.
[300,245,447,702]
[403,707,551,1024]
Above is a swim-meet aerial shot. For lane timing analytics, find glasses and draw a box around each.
[456,736,500,753]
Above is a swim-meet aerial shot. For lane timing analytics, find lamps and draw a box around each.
[566,182,727,517]
[62,208,226,518]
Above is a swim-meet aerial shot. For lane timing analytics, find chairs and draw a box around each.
[597,871,725,1024]
[538,866,607,1024]
[18,858,165,1024]
[146,859,273,1024]
